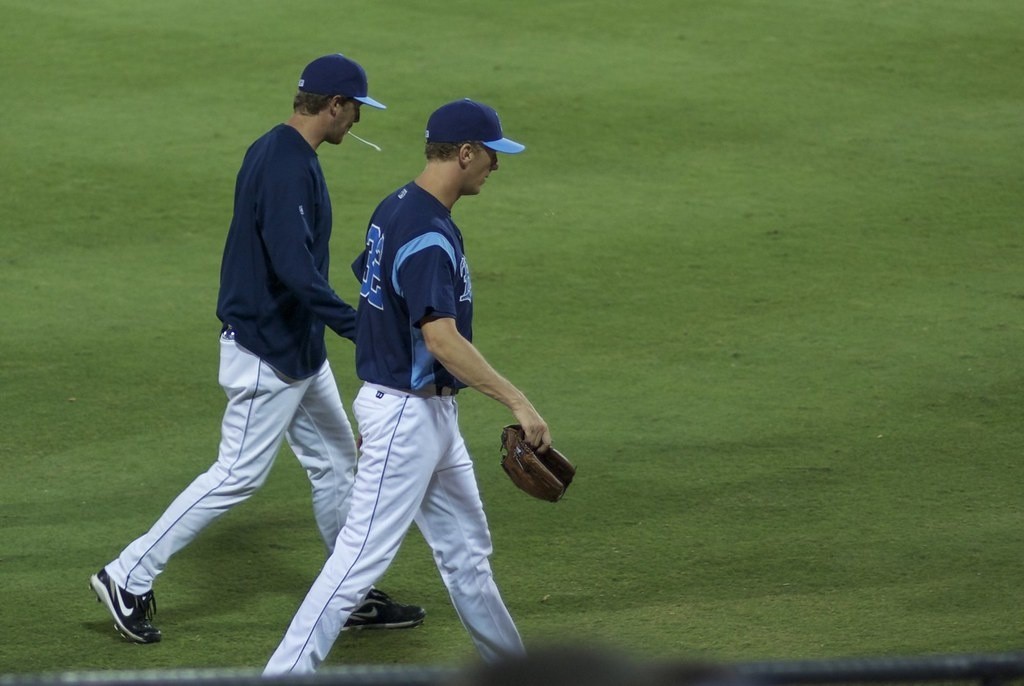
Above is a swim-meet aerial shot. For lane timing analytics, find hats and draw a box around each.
[425,98,525,154]
[297,53,387,110]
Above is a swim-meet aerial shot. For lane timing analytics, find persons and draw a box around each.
[260,97,553,676]
[88,54,426,644]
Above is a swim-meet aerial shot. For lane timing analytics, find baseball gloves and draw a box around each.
[498,423,579,503]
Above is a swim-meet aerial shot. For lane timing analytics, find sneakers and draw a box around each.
[88,570,162,645]
[340,588,425,631]
